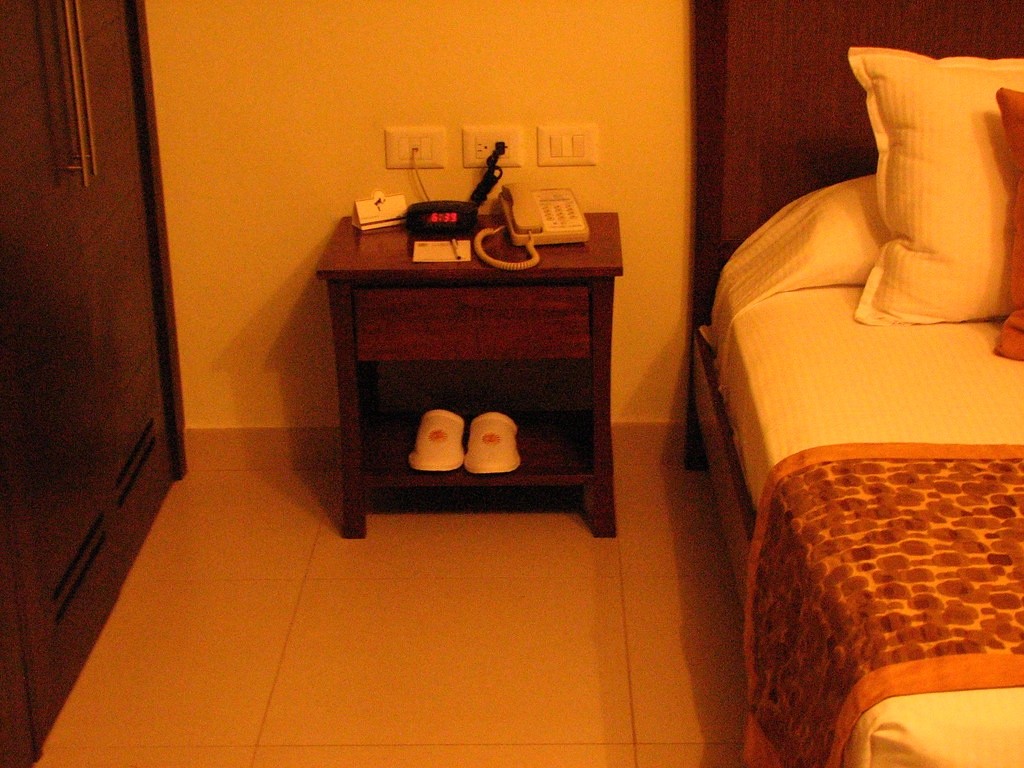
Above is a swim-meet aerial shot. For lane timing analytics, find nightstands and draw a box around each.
[315,209,624,539]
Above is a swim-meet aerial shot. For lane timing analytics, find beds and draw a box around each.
[685,0,1023,767]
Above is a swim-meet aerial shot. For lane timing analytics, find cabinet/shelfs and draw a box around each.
[0,0,190,768]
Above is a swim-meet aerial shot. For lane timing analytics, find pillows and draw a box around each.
[845,45,1023,322]
[699,167,881,396]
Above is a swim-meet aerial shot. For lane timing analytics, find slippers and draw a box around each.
[463,411,521,474]
[408,409,465,472]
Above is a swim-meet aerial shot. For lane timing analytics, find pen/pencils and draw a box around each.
[451,238,461,259]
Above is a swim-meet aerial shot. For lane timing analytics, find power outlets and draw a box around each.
[463,126,524,168]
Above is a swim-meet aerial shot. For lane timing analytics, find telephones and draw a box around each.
[499,183,590,246]
